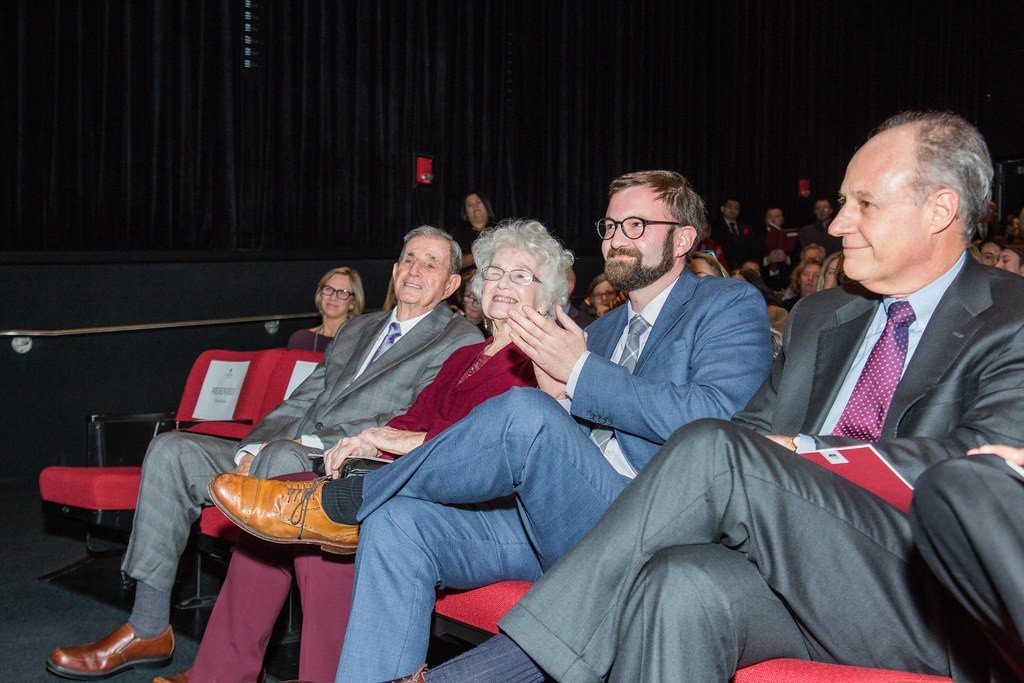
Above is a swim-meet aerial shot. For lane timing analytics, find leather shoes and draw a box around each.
[46,623,174,680]
[154,668,193,683]
[206,473,359,555]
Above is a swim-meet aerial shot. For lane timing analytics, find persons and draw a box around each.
[377,109,1024,683]
[909,443,1024,683]
[43,224,486,683]
[452,184,1024,379]
[205,170,772,683]
[189,220,574,683]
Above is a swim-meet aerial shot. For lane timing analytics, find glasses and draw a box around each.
[462,295,478,304]
[703,250,717,257]
[592,290,615,299]
[320,284,354,300]
[481,266,542,286]
[596,217,680,239]
[816,206,831,211]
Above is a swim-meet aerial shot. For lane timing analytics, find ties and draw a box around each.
[588,315,650,455]
[366,322,401,371]
[832,301,916,444]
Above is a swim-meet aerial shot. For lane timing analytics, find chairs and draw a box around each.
[37,349,957,683]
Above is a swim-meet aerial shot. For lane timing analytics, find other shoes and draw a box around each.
[385,664,428,683]
